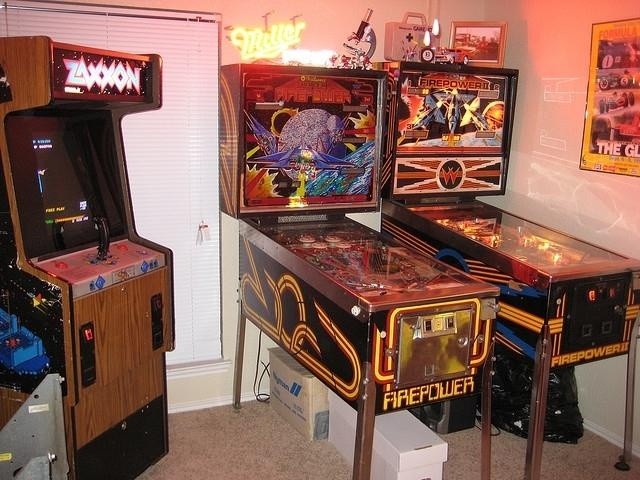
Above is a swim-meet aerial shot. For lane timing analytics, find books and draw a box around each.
[268,346,330,443]
[327,387,450,479]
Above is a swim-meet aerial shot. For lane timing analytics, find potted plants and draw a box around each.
[448,19,508,68]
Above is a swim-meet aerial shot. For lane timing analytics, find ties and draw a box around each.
[4,112,128,259]
